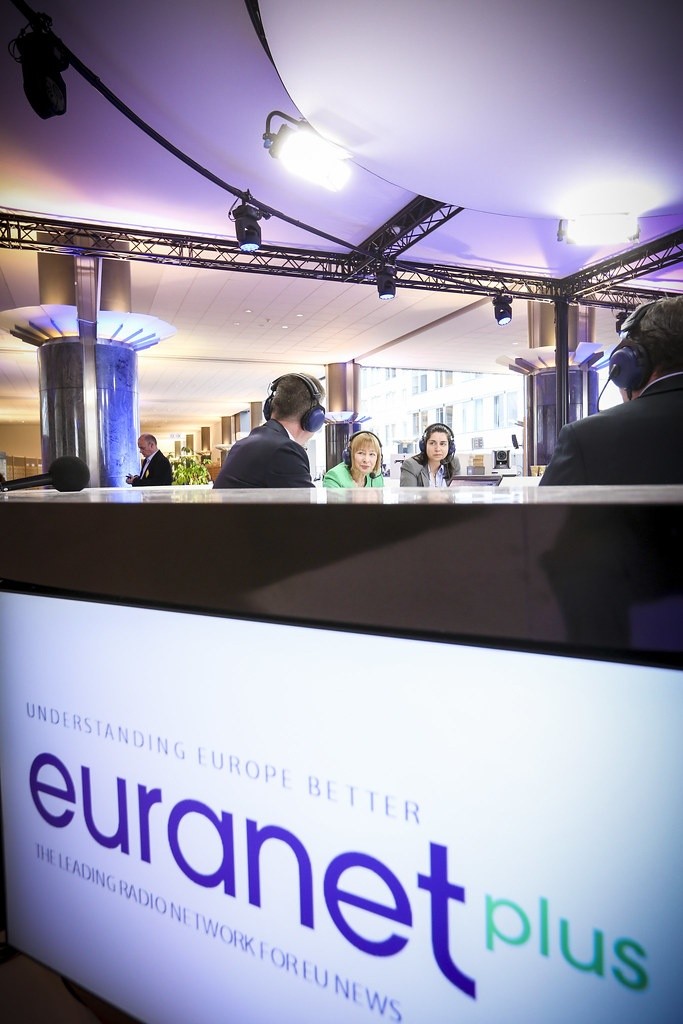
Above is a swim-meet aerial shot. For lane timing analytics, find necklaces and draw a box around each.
[431,471,437,477]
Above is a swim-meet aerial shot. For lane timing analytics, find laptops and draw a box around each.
[447,475,502,488]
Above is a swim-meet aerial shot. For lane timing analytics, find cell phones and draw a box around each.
[126,476,131,478]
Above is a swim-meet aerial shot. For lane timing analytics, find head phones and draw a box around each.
[341,430,383,466]
[418,423,455,455]
[608,301,657,400]
[262,373,325,432]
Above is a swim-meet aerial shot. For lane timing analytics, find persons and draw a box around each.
[323,430,384,488]
[399,423,460,487]
[537,295,683,487]
[212,372,326,489]
[126,434,172,487]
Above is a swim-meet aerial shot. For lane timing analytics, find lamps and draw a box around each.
[262,110,353,192]
[556,214,641,245]
[232,205,271,252]
[376,266,397,299]
[18,31,71,119]
[492,296,513,325]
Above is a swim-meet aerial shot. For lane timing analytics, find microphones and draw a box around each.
[0,456,90,493]
[439,454,454,465]
[369,466,382,479]
